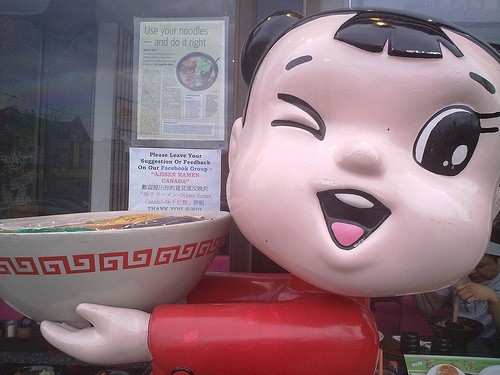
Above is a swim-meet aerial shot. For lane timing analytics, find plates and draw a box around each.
[479,365,500,375]
[426,363,465,375]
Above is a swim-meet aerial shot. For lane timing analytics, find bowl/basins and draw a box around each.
[1,209,234,325]
[176,52,218,91]
[429,315,484,343]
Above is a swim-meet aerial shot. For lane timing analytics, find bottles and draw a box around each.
[400,331,455,361]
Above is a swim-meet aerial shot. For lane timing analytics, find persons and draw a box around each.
[414,227,500,335]
[38,5,498,375]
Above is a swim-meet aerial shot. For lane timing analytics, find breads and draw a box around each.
[433,364,459,375]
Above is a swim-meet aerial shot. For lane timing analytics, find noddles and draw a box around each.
[177,53,219,92]
[431,279,485,343]
[0,206,232,329]
